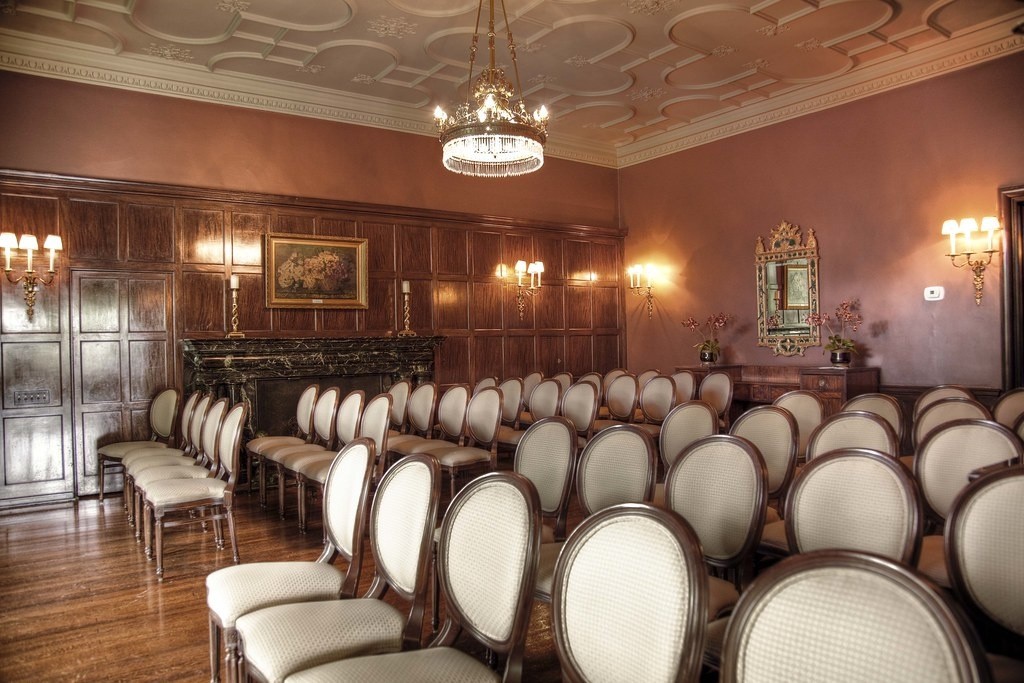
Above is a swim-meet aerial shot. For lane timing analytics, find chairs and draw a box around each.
[96,366,1024,683]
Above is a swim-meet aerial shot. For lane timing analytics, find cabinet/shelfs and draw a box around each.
[675,365,881,420]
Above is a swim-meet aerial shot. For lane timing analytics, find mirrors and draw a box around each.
[754,219,822,357]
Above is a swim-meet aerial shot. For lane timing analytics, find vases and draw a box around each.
[700,350,715,365]
[829,350,851,367]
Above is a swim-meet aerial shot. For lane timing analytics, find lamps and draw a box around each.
[433,0,550,178]
[628,263,653,318]
[941,217,1003,306]
[0,232,63,321]
[514,260,544,321]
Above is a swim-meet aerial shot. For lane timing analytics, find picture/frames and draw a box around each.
[265,232,369,310]
[784,263,809,310]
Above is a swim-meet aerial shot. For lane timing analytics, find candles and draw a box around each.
[231,275,239,288]
[403,280,410,292]
[775,290,779,298]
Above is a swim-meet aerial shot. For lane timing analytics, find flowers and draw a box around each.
[806,297,863,358]
[679,312,734,361]
